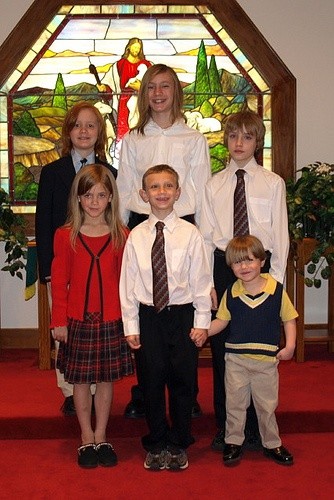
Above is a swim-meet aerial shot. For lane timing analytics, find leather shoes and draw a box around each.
[263,445,293,464]
[222,444,241,464]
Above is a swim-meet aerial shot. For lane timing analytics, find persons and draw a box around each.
[200,108,290,453]
[190,235,300,464]
[35,103,118,419]
[115,63,212,419]
[118,163,213,471]
[49,164,135,468]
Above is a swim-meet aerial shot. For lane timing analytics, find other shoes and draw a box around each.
[212,430,225,449]
[64,396,97,416]
[96,441,117,467]
[124,402,145,418]
[77,443,97,468]
[191,399,202,417]
[242,430,261,449]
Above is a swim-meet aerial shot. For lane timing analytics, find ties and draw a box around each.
[80,158,87,168]
[151,221,169,312]
[233,169,250,240]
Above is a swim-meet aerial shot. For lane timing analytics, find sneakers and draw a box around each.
[144,449,166,470]
[166,448,189,470]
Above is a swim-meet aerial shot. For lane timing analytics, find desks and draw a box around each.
[26,237,318,371]
